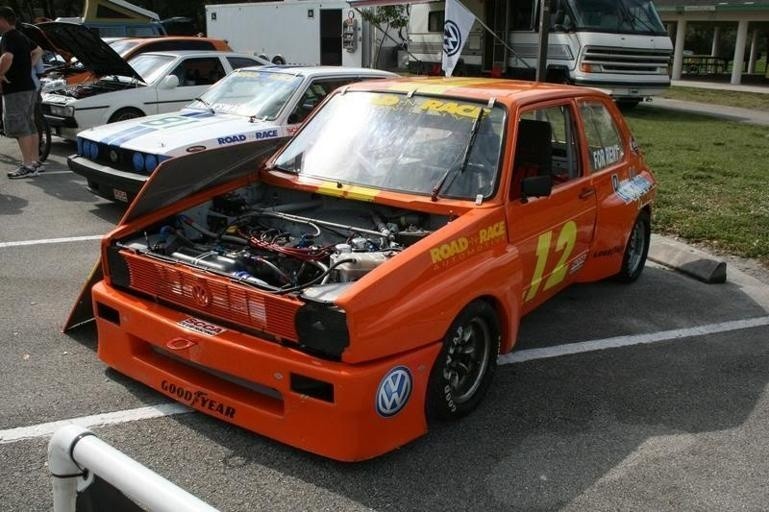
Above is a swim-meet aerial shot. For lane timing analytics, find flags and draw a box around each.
[442,0,475,79]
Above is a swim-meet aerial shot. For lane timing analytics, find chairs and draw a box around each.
[508,120,554,202]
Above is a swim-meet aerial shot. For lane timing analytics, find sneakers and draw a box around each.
[7,160,45,179]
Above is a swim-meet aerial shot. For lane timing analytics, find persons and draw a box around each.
[0,6,45,179]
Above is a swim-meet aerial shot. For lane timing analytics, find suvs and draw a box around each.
[21,17,234,94]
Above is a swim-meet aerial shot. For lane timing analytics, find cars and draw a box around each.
[39,50,277,141]
[91,76,657,462]
[406,0,674,112]
[67,65,400,208]
